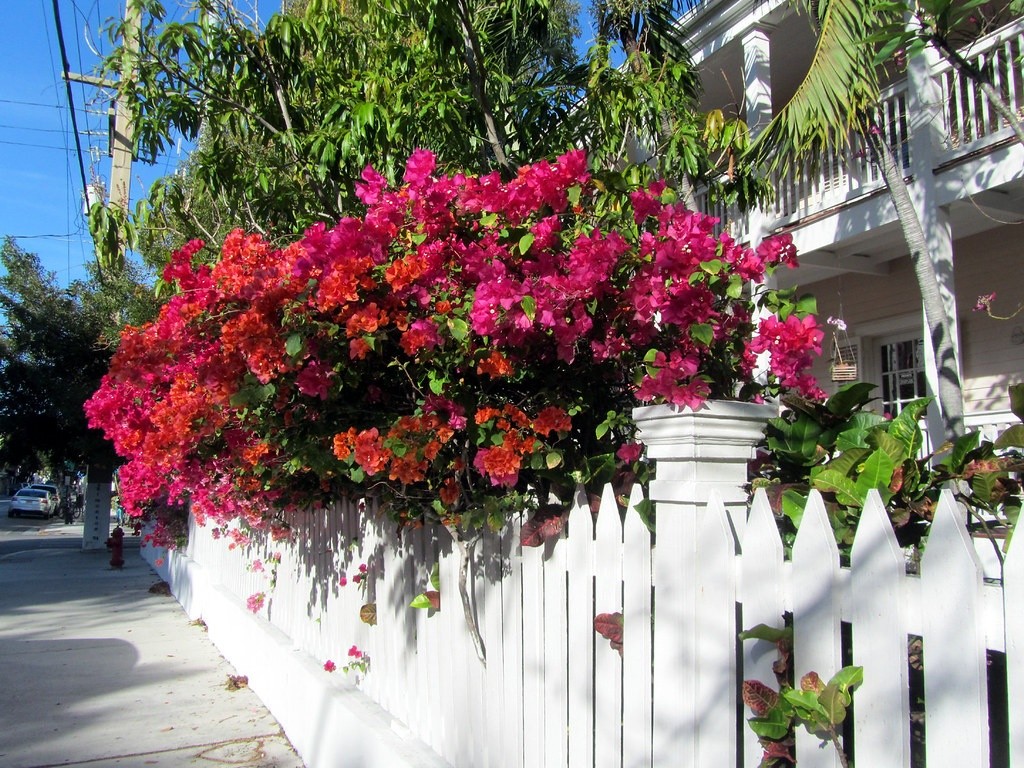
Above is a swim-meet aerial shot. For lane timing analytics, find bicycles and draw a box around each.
[56,499,81,518]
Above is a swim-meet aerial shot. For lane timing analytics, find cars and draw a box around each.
[30,484,62,513]
[7,488,58,520]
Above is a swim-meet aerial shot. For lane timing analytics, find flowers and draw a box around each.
[825,317,847,364]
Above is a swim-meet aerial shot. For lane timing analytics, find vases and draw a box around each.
[830,362,858,381]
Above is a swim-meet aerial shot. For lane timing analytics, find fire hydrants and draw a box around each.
[105,525,127,570]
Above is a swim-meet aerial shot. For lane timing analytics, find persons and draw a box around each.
[73,475,116,515]
